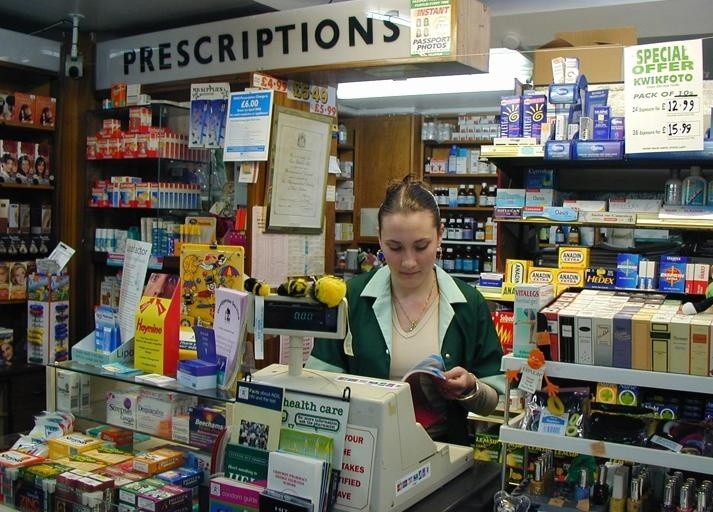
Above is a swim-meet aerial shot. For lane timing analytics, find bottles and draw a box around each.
[509,388,526,410]
[666,163,713,207]
[529,449,712,511]
[335,248,385,271]
[537,227,607,246]
[423,145,495,274]
[338,120,347,143]
[421,119,456,142]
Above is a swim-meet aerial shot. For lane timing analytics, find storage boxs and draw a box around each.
[532,23,639,85]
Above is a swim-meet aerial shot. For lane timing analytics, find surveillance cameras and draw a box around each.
[65,52,83,78]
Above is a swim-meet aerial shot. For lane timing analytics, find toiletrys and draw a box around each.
[528,451,713,512]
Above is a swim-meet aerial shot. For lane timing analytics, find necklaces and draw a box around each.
[391,276,436,331]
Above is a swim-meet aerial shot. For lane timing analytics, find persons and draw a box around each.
[31,157,51,186]
[302,173,508,447]
[10,263,27,288]
[0,264,10,285]
[0,154,15,184]
[5,95,16,121]
[16,156,34,184]
[1,336,13,366]
[143,273,178,299]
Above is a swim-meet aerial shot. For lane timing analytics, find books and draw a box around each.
[400,354,448,410]
[208,400,333,512]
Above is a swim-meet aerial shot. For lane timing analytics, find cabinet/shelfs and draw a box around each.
[83,101,212,262]
[469,139,713,512]
[42,354,246,495]
[0,61,88,441]
[336,110,496,280]
